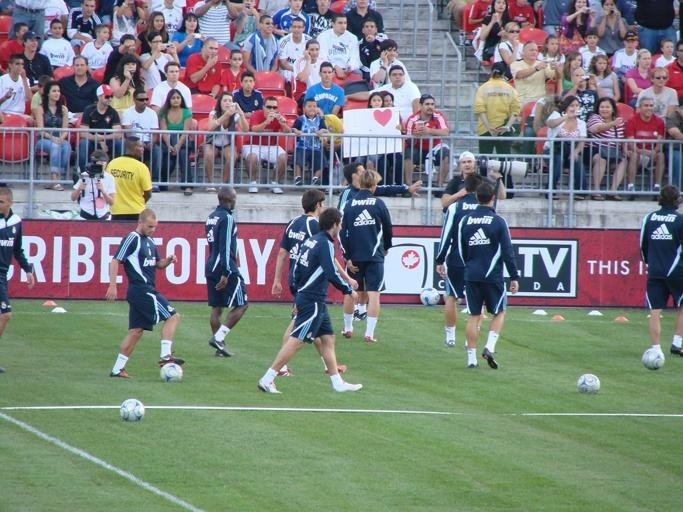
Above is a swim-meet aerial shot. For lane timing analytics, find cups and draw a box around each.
[653,75,668,79]
[136,97,149,102]
[506,30,520,33]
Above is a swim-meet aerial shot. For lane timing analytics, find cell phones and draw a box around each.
[478,159,529,179]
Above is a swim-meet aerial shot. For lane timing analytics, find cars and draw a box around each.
[415,122,424,133]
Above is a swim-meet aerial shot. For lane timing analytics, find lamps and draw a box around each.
[278,114,298,160]
[536,7,542,29]
[92,66,145,84]
[178,67,190,86]
[0,15,12,44]
[519,101,538,138]
[253,71,287,99]
[218,46,233,72]
[463,3,481,60]
[197,119,217,159]
[536,127,548,154]
[343,98,370,110]
[0,115,35,160]
[508,78,515,87]
[616,103,635,122]
[54,67,74,80]
[333,75,363,85]
[188,118,202,161]
[236,117,251,159]
[519,28,549,53]
[191,93,216,119]
[274,97,300,114]
[474,28,493,86]
[546,79,556,94]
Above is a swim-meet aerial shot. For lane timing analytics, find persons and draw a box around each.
[106,209,185,378]
[257,209,363,393]
[442,151,506,209]
[206,185,249,357]
[457,183,519,369]
[0,187,34,373]
[0,0,449,196]
[337,163,423,322]
[447,0,683,202]
[271,188,348,376]
[639,187,683,356]
[436,173,483,346]
[336,170,393,343]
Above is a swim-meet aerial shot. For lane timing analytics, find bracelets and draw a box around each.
[215,120,220,126]
[535,67,539,71]
[380,66,386,70]
[565,114,569,120]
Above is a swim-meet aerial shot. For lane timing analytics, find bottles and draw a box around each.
[22,31,42,41]
[459,151,475,162]
[95,83,114,96]
[492,61,506,75]
[624,30,638,41]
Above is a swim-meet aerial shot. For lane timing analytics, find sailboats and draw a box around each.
[577,374,600,394]
[120,399,144,421]
[643,348,665,370]
[420,287,440,305]
[160,363,183,384]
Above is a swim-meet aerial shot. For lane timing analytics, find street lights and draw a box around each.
[600,145,623,162]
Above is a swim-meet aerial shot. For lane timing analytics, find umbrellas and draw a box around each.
[184,191,193,196]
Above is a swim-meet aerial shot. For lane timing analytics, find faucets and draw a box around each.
[574,193,586,201]
[545,192,559,200]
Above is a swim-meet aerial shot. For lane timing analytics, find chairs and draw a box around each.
[467,359,479,369]
[351,309,364,322]
[651,187,661,201]
[158,353,185,368]
[332,381,364,393]
[324,364,347,376]
[276,368,295,377]
[627,186,636,201]
[359,311,368,320]
[248,180,260,193]
[311,177,321,186]
[215,348,234,358]
[294,176,304,185]
[110,367,132,378]
[219,184,236,194]
[445,339,456,348]
[206,186,217,194]
[670,343,683,357]
[269,181,285,194]
[482,347,498,370]
[434,191,443,198]
[364,335,378,343]
[257,377,283,394]
[208,335,232,357]
[340,328,353,338]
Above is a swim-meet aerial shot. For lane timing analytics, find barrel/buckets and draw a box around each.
[591,193,606,201]
[51,182,65,191]
[606,193,623,201]
